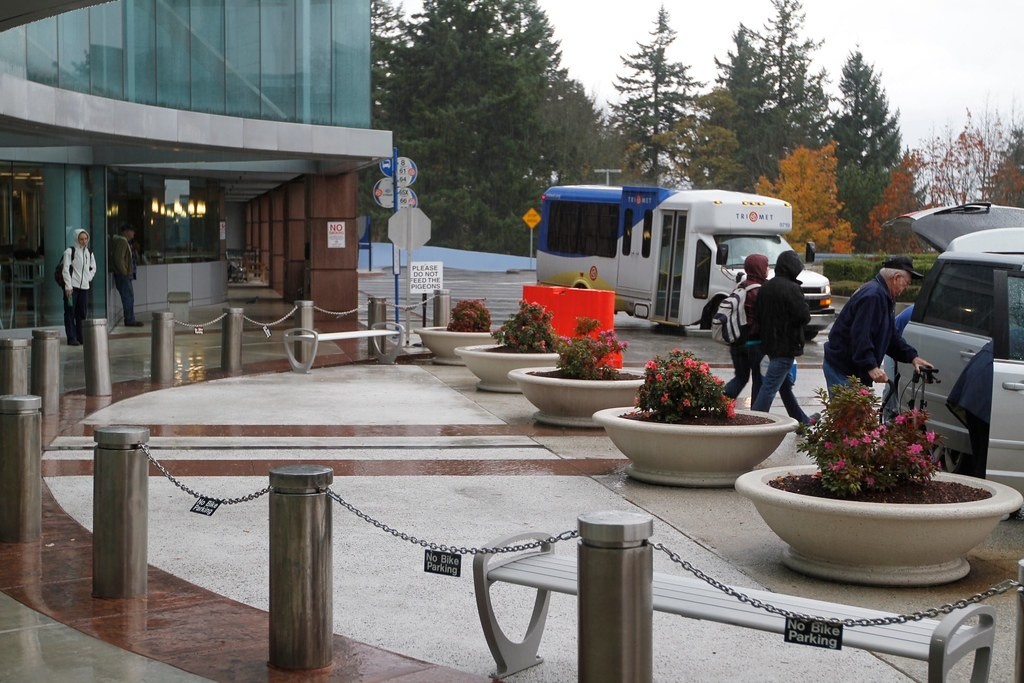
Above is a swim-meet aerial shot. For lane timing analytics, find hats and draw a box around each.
[882,255,925,279]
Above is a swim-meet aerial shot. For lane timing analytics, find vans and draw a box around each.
[893,204,1024,499]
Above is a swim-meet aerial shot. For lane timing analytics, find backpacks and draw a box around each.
[55,246,76,289]
[711,281,762,344]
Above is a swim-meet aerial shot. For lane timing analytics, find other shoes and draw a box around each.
[69,341,80,346]
[796,412,820,434]
[125,321,144,327]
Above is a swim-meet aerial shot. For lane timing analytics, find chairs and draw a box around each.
[0,258,45,331]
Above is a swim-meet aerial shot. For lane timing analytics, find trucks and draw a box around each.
[535,185,836,341]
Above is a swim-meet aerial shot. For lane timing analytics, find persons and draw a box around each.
[721,253,770,411]
[112,223,144,327]
[823,255,935,416]
[945,326,1024,520]
[751,250,821,435]
[13,235,44,278]
[62,229,97,346]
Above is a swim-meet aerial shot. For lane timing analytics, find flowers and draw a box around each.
[554,316,628,380]
[628,345,736,420]
[490,294,561,353]
[794,375,948,493]
[448,297,492,333]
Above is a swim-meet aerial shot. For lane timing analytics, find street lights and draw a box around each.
[594,168,621,186]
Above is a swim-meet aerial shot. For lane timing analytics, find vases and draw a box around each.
[413,326,506,367]
[735,466,1024,588]
[506,365,649,427]
[454,344,563,396]
[592,406,800,489]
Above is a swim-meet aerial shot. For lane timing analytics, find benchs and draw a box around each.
[471,530,997,683]
[282,322,397,373]
